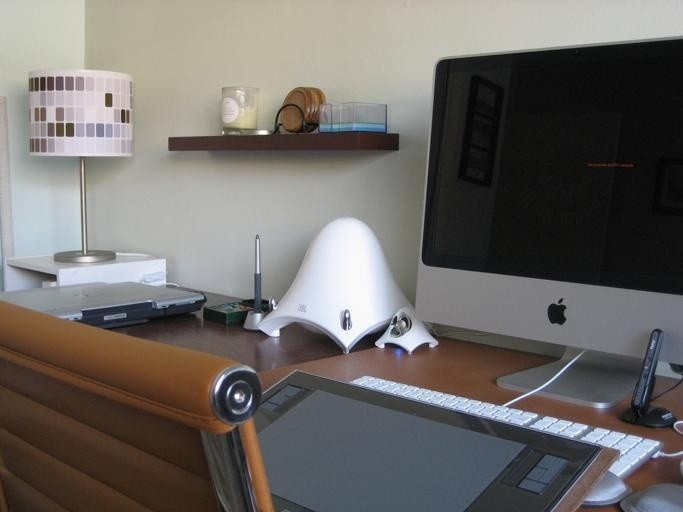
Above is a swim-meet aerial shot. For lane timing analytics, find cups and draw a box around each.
[219,86,259,136]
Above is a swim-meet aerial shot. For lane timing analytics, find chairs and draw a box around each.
[0,302,273,512]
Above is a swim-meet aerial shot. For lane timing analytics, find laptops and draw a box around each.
[1,278,207,324]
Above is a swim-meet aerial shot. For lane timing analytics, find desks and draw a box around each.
[5,284,683,511]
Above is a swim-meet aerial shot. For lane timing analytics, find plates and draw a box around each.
[280,87,327,133]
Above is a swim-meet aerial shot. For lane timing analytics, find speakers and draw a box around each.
[376,304,438,356]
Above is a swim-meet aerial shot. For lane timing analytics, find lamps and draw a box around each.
[25,67,137,263]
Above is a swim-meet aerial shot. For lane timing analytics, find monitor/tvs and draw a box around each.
[413,33,683,408]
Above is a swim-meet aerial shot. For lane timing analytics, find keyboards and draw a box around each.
[349,372,662,480]
